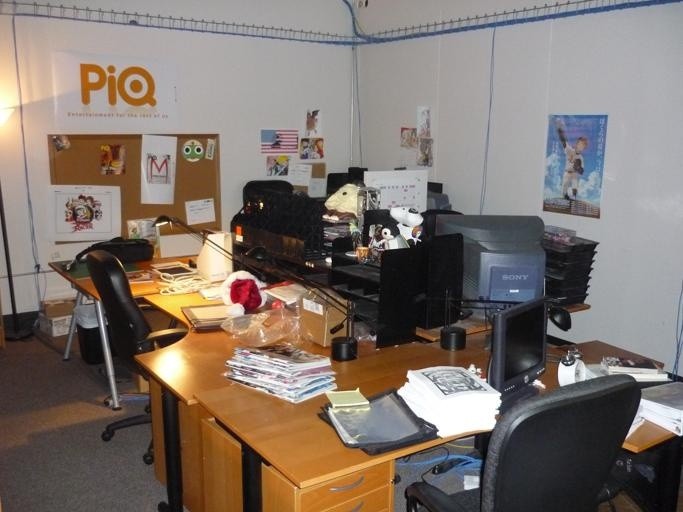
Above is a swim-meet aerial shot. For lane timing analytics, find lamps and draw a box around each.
[151,215,266,280]
[245,245,359,321]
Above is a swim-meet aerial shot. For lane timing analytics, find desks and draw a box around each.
[133,281,495,512]
[47,255,198,409]
[193,327,683,512]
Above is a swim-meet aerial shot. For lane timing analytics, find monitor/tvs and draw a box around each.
[489,294,549,415]
[426,190,452,210]
[434,213,547,320]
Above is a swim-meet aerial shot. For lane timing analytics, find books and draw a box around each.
[396,364,503,439]
[221,345,338,403]
[259,280,307,305]
[181,302,231,327]
[601,356,682,436]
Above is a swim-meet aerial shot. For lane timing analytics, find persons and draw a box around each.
[556,118,588,208]
[300,138,324,158]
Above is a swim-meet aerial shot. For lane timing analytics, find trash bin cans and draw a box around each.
[72,305,108,364]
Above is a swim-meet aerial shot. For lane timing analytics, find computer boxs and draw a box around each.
[612,437,683,511]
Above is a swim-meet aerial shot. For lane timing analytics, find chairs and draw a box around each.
[87,249,187,463]
[405,374,641,512]
